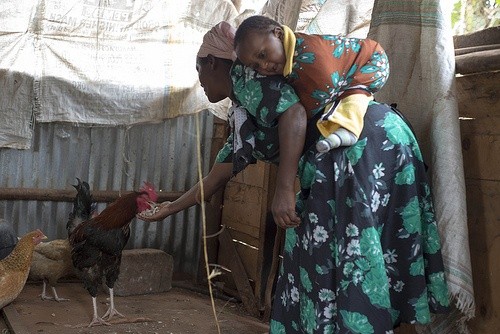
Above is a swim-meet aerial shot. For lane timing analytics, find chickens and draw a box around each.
[66,177,159,328]
[17,212,99,303]
[0,229,49,311]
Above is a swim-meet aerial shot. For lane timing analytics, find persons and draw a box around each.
[135,22,449,333]
[234,16,390,153]
